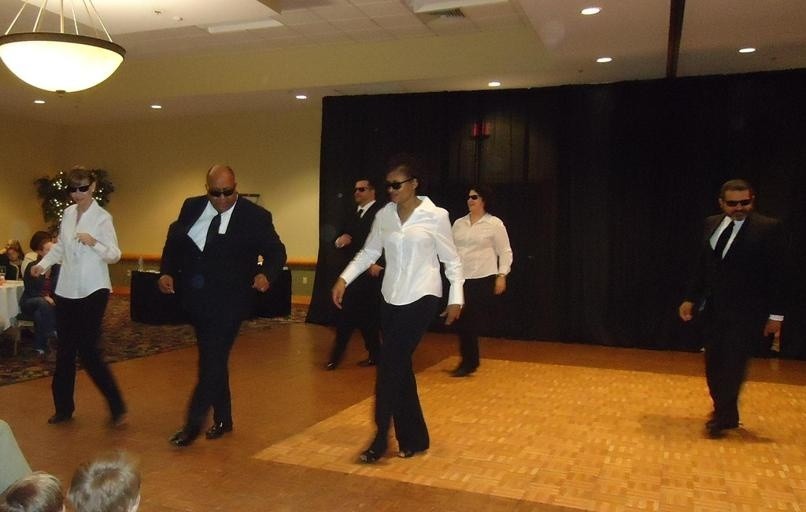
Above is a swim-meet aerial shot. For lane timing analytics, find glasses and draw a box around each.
[352,185,373,193]
[383,175,417,188]
[67,182,94,194]
[722,195,754,207]
[465,194,487,201]
[206,185,236,197]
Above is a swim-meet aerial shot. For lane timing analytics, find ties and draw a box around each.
[202,211,223,257]
[713,218,736,258]
[354,208,362,221]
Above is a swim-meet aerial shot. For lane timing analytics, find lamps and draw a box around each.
[0,0,126,93]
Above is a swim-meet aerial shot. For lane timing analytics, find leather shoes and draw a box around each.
[356,355,377,367]
[704,409,740,436]
[44,410,76,423]
[452,355,480,375]
[106,414,128,428]
[205,421,233,440]
[359,441,389,462]
[395,447,421,457]
[167,424,202,446]
[322,357,341,370]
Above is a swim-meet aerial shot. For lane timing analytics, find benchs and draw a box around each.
[130,262,292,325]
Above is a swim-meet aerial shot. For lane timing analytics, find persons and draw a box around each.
[677,179,792,436]
[447,186,512,377]
[157,164,288,446]
[5,248,23,279]
[30,168,128,427]
[324,179,390,370]
[0,470,62,511]
[21,230,49,278]
[19,240,63,358]
[331,154,465,463]
[65,449,143,510]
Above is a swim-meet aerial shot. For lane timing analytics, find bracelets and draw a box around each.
[497,274,505,278]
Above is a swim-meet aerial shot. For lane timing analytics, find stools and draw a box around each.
[13,320,52,358]
